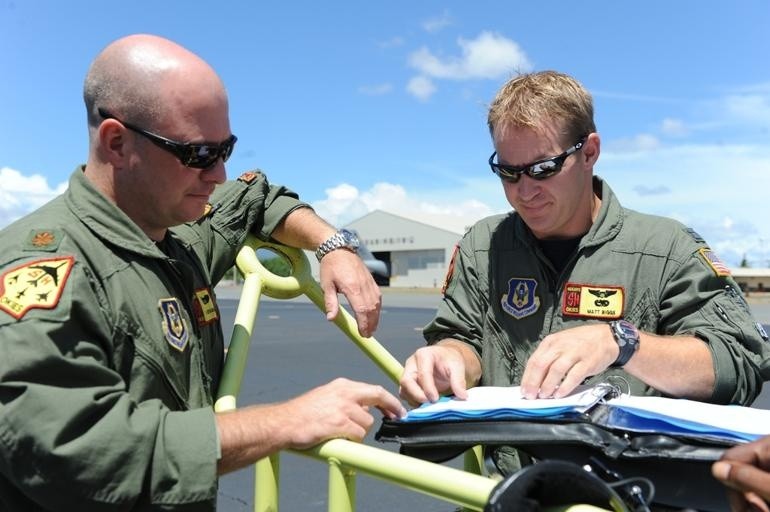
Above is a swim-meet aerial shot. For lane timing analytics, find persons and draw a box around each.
[710,432,770,512]
[396,70,769,512]
[2,33,408,512]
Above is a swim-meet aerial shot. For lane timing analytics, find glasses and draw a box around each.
[488,137,586,183]
[98,107,238,169]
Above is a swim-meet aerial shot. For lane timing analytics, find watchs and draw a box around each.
[608,311,642,371]
[313,226,365,260]
